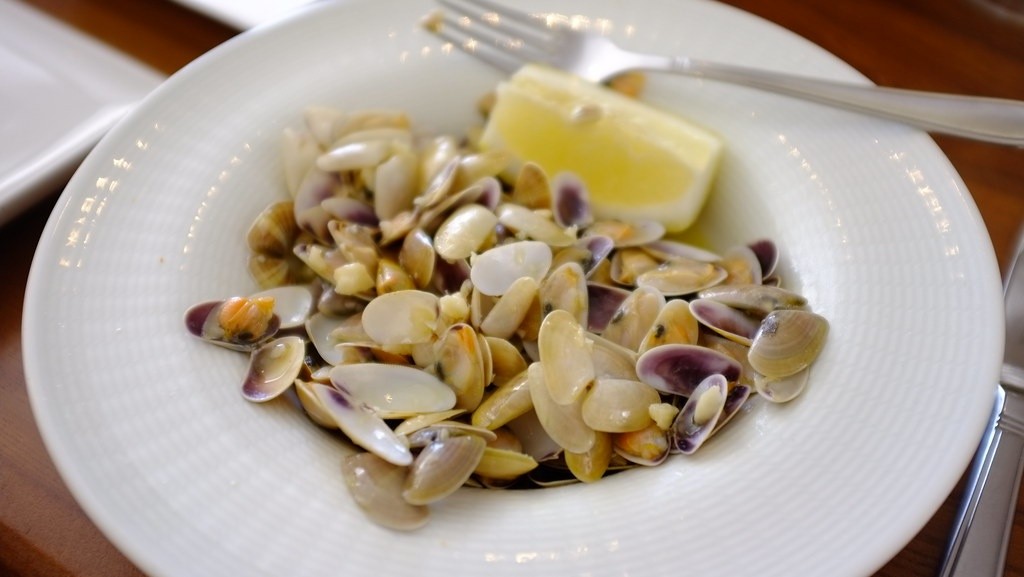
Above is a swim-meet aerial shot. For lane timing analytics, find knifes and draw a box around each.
[935,217,1024,573]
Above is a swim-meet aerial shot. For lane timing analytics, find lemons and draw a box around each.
[480,65,722,232]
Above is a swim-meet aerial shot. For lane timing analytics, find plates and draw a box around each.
[0,0,169,230]
[21,0,1005,577]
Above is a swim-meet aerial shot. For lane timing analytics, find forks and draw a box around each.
[425,0,1024,147]
